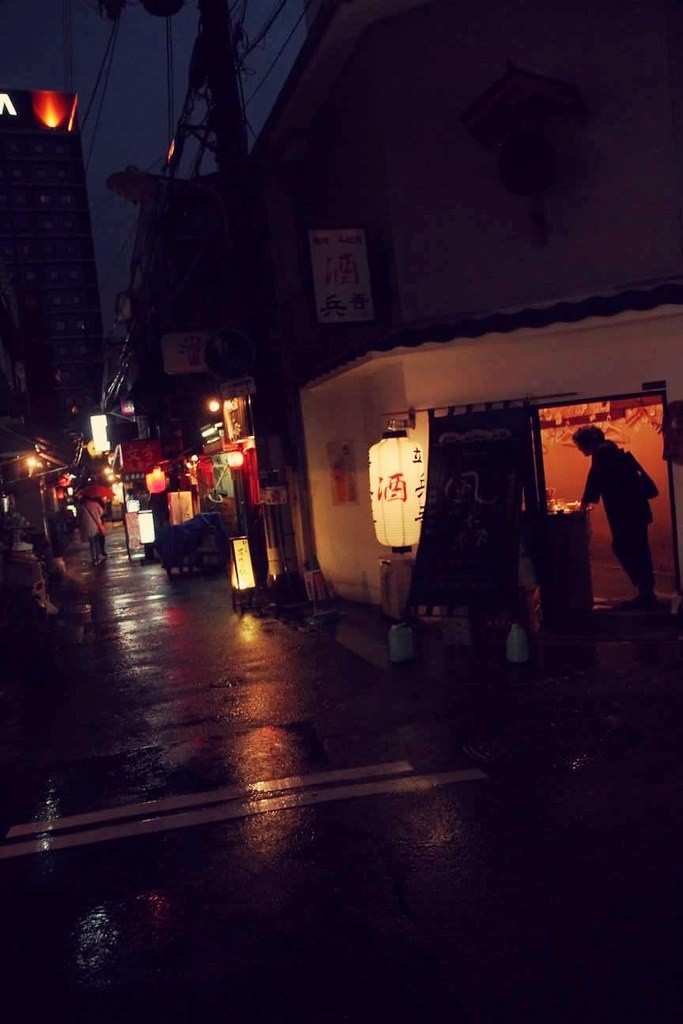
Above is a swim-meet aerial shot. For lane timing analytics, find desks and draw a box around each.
[546,506,596,612]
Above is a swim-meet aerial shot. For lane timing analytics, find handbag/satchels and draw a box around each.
[625,450,659,500]
[99,526,107,536]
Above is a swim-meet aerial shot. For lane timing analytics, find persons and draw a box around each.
[570,424,658,610]
[79,495,107,565]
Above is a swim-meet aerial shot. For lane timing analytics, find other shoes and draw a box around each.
[617,591,656,609]
[100,548,108,557]
[93,557,100,566]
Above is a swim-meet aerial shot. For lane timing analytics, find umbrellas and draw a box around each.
[80,484,114,500]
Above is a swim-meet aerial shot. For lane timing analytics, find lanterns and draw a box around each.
[145,468,166,493]
[367,432,429,553]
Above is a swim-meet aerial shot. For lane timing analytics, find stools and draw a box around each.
[231,587,260,614]
